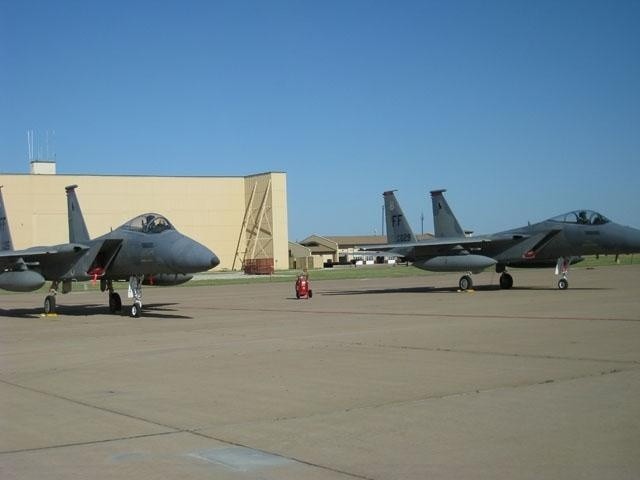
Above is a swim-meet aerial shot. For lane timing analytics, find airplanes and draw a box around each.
[341,189,640,292]
[0,184,220,318]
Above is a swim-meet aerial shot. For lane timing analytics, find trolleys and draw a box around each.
[294,272,314,300]
